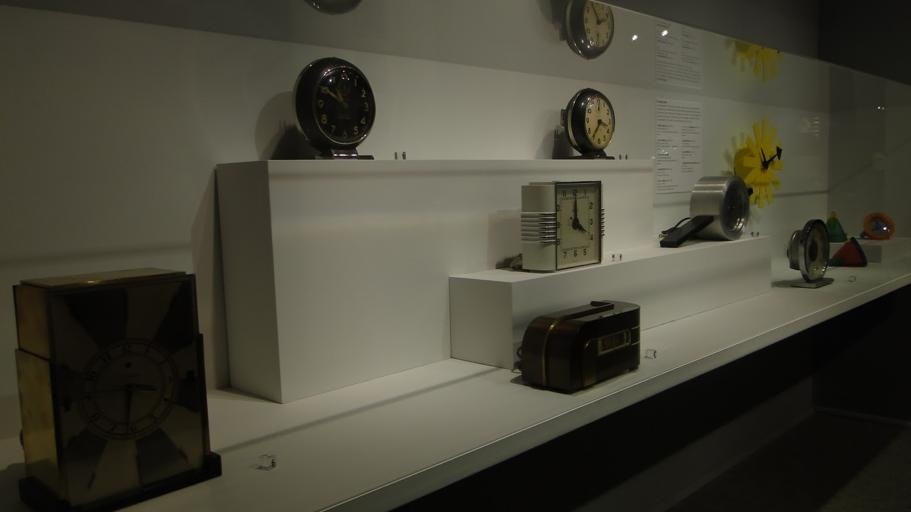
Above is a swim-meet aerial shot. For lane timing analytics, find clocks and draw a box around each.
[14,265,223,512]
[519,178,605,273]
[295,58,378,160]
[863,213,894,240]
[788,217,831,284]
[564,88,617,159]
[690,174,751,240]
[563,1,616,62]
